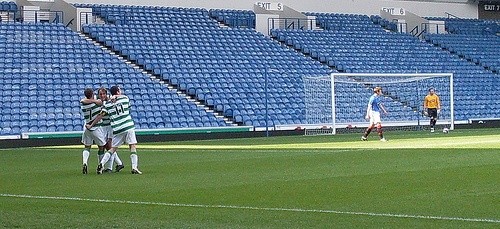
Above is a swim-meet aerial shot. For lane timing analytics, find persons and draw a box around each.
[425,88,440,132]
[361,87,387,141]
[85,86,143,175]
[81,88,107,174]
[80,88,128,174]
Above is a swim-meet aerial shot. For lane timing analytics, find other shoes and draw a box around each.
[96,164,103,176]
[381,138,388,142]
[132,169,143,175]
[105,169,113,174]
[115,166,125,173]
[362,137,367,141]
[431,128,435,133]
[83,164,89,176]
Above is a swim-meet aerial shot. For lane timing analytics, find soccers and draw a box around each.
[442,127,450,134]
